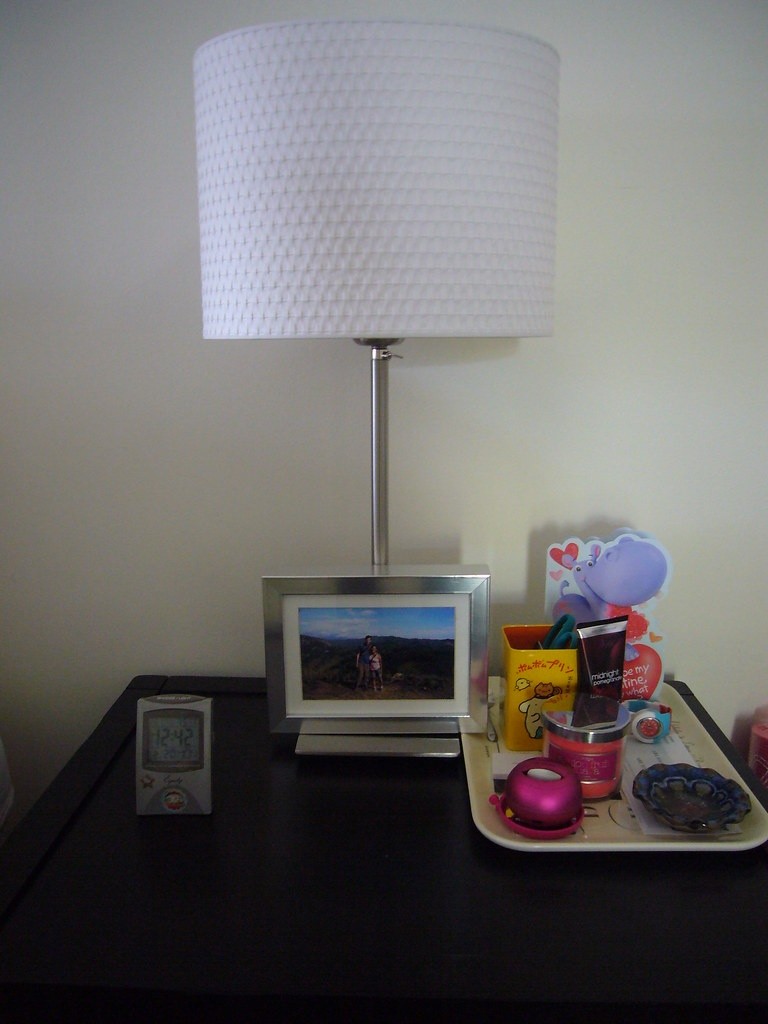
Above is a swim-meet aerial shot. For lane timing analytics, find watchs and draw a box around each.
[622,698,673,743]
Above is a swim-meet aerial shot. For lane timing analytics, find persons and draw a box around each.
[354,635,384,690]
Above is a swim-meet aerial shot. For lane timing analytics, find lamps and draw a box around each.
[190,18,567,568]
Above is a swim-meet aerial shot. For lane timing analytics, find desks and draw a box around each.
[0,675,768,1023]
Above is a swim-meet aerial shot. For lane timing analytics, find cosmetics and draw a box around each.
[539,615,628,800]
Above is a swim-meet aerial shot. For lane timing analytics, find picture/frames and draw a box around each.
[261,573,490,738]
